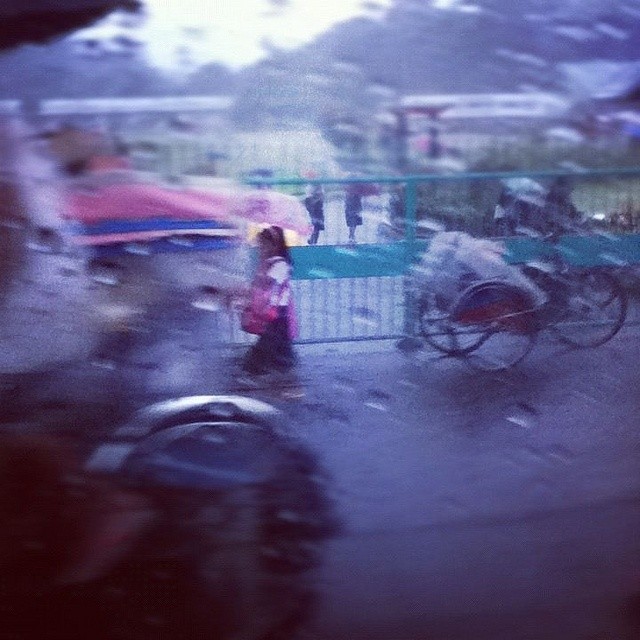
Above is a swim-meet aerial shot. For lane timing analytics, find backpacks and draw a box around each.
[241,259,291,335]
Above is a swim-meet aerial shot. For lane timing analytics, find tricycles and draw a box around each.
[412,220,628,374]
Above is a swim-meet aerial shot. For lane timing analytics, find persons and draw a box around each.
[303,181,326,244]
[233,224,301,388]
[344,184,366,244]
